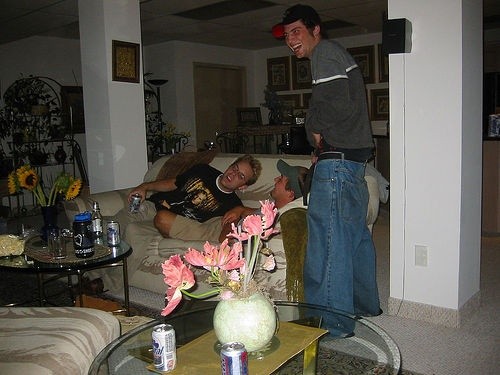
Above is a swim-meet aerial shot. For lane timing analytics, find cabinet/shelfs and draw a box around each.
[0,76,87,192]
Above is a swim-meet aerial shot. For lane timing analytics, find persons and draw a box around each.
[271,4,383,337]
[72,155,262,296]
[153,159,311,247]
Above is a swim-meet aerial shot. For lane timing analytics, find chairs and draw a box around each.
[216,131,249,153]
[279,127,314,155]
[155,134,188,157]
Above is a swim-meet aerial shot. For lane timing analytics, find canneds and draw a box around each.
[129,193,141,214]
[152,324,176,373]
[220,341,249,375]
[106,221,120,246]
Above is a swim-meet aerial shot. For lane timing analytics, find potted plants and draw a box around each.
[260,87,289,127]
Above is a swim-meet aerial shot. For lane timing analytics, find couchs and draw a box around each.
[63,153,389,321]
[0,307,121,375]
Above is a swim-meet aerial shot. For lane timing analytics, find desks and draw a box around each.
[234,126,288,155]
[0,228,134,317]
[88,302,402,375]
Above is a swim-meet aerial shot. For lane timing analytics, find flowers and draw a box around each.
[7,164,82,207]
[160,199,279,317]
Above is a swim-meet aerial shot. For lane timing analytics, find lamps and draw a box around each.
[144,71,168,133]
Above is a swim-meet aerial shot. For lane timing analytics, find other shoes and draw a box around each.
[83,278,109,296]
[288,317,354,341]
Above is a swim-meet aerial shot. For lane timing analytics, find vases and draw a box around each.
[40,205,60,245]
[213,290,276,352]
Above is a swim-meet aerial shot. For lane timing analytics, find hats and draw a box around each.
[271,4,317,38]
[276,159,302,199]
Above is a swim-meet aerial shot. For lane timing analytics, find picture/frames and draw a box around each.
[267,43,389,120]
[61,86,84,134]
[112,40,140,83]
[236,107,262,126]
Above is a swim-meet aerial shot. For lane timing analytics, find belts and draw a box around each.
[302,152,373,206]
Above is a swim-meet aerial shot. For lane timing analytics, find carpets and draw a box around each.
[127,330,430,375]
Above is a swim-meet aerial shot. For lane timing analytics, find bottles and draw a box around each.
[72,210,95,258]
[91,202,103,242]
[54,146,66,162]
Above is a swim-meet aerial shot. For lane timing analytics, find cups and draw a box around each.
[46,236,66,260]
